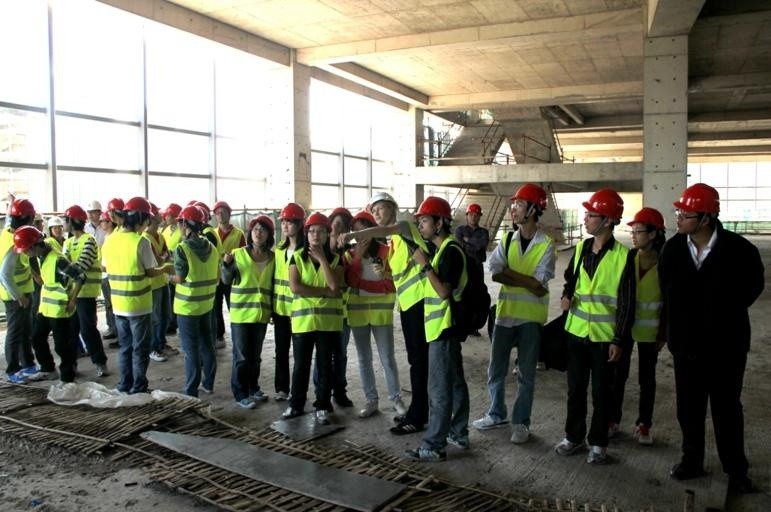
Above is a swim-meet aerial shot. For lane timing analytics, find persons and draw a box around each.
[341,210,407,417]
[313,207,352,408]
[472,183,559,443]
[609,207,667,445]
[282,211,345,425]
[554,190,637,464]
[456,204,489,337]
[221,216,275,408]
[404,196,470,462]
[1,196,246,403]
[272,203,307,400]
[337,191,436,436]
[658,183,764,499]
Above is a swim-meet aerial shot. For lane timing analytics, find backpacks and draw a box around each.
[436,241,490,338]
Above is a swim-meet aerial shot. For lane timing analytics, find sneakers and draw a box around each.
[671,463,703,480]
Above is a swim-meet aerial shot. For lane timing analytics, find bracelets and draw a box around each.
[421,263,433,274]
